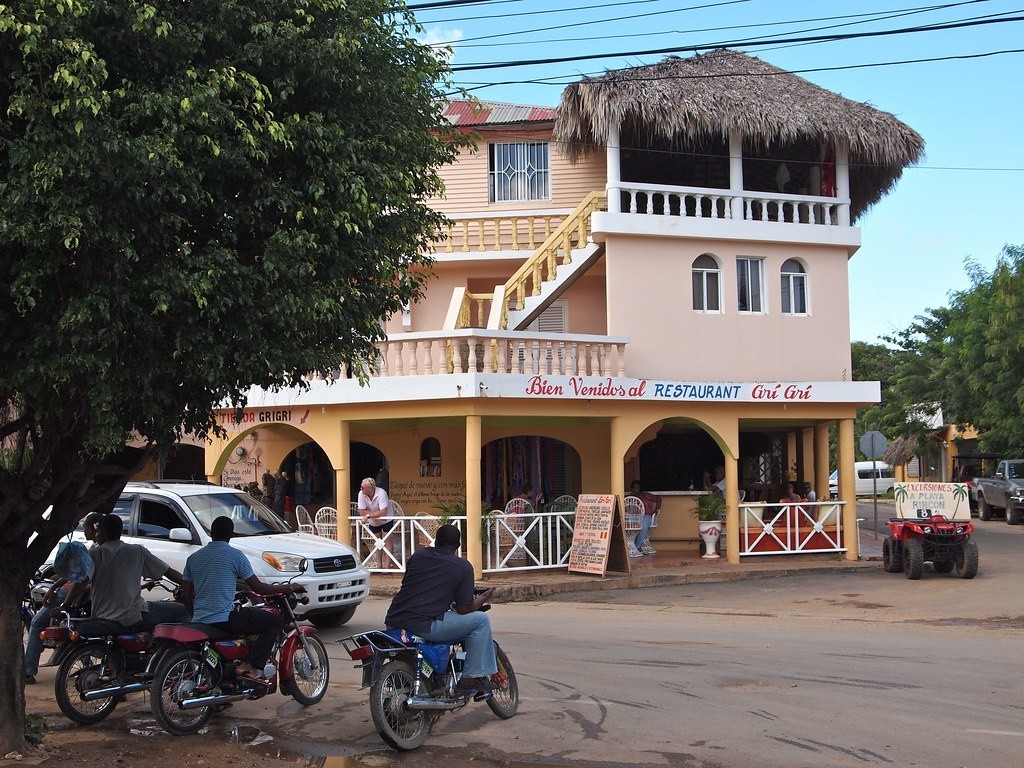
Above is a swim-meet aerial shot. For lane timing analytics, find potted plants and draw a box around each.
[688,486,726,559]
[431,498,494,567]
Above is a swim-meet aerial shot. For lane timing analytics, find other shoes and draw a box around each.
[455,676,497,696]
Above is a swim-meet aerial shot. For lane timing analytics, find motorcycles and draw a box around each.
[335,586,519,751]
[883,509,979,580]
[21,560,330,735]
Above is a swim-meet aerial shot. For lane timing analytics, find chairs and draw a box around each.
[294,500,443,569]
[138,507,170,539]
[485,494,661,569]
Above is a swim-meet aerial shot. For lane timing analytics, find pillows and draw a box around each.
[780,499,808,528]
[738,502,766,528]
[817,498,843,526]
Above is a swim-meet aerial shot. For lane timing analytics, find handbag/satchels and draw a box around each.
[55,533,91,584]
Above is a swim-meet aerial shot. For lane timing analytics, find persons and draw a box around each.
[384,525,498,693]
[510,483,543,530]
[783,482,816,517]
[625,480,662,555]
[23,514,183,684]
[183,516,306,689]
[234,463,275,509]
[358,477,394,575]
[274,471,293,516]
[703,466,740,506]
[375,469,389,497]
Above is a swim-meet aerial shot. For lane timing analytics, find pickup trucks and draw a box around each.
[970,459,1024,525]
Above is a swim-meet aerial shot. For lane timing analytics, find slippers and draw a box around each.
[236,667,270,687]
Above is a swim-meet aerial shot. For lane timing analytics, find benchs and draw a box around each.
[738,525,843,552]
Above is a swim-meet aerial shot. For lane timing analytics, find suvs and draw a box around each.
[947,464,982,507]
[28,480,371,626]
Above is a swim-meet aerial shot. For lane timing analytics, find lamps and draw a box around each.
[255,446,263,466]
[235,446,246,456]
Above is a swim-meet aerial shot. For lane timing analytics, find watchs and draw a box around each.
[366,513,370,519]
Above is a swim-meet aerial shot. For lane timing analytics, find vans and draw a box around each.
[829,461,895,499]
